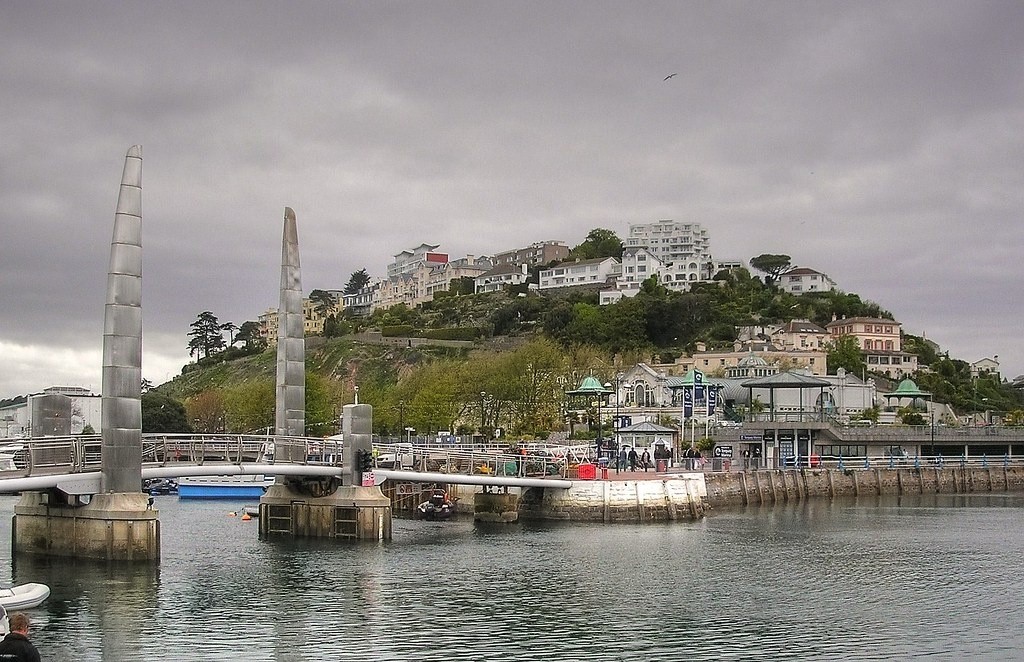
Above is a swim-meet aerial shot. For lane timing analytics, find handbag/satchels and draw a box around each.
[521,450,526,454]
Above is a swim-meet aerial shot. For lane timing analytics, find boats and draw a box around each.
[0,582,50,612]
[241,504,260,517]
[176,475,277,500]
[418,494,451,522]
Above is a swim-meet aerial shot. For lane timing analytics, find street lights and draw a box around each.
[605,377,621,474]
[399,398,404,443]
[480,392,493,453]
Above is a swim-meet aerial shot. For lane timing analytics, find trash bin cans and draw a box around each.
[713,458,723,472]
[656,459,668,472]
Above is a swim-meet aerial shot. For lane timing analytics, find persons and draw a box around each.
[641,448,650,472]
[755,394,763,412]
[0,612,41,662]
[619,447,627,472]
[897,449,908,468]
[628,447,639,472]
[743,445,760,457]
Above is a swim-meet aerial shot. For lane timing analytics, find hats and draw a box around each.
[645,448,647,450]
